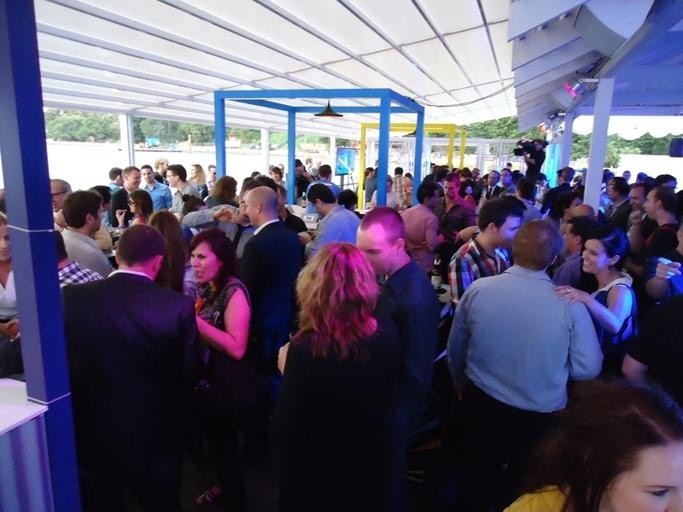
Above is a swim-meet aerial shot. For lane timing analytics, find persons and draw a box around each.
[190,229,252,361]
[62,190,112,276]
[206,165,216,190]
[112,166,142,229]
[355,207,433,508]
[187,164,208,200]
[295,159,314,198]
[305,183,360,254]
[139,165,172,212]
[514,179,542,225]
[274,184,308,233]
[60,223,195,512]
[448,196,522,310]
[108,167,123,198]
[54,230,104,291]
[50,179,71,212]
[149,211,190,293]
[502,379,683,512]
[89,185,113,227]
[539,167,575,218]
[604,168,677,189]
[154,158,169,185]
[256,175,308,233]
[644,224,683,300]
[545,204,595,279]
[424,162,548,198]
[548,193,583,223]
[0,211,24,378]
[447,221,603,435]
[400,183,444,282]
[552,216,598,295]
[627,182,658,238]
[205,176,238,208]
[624,187,678,276]
[181,176,276,241]
[523,138,546,177]
[306,164,339,214]
[434,174,476,249]
[241,163,287,188]
[165,164,201,222]
[363,167,414,211]
[239,187,305,345]
[622,294,683,393]
[604,180,630,229]
[115,190,153,236]
[554,225,639,352]
[279,242,401,511]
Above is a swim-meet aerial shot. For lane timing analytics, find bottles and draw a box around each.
[301,191,307,208]
[430,252,443,290]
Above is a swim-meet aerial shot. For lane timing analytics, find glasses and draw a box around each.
[166,174,174,178]
[50,191,65,197]
[127,199,135,205]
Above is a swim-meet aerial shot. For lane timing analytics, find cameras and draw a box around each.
[515,139,539,155]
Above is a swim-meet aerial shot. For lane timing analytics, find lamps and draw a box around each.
[314,101,343,117]
[562,74,599,97]
[545,109,566,120]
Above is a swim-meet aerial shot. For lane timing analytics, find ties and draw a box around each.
[232,223,253,256]
[489,188,492,194]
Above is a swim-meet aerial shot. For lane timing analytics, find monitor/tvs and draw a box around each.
[336,147,358,175]
